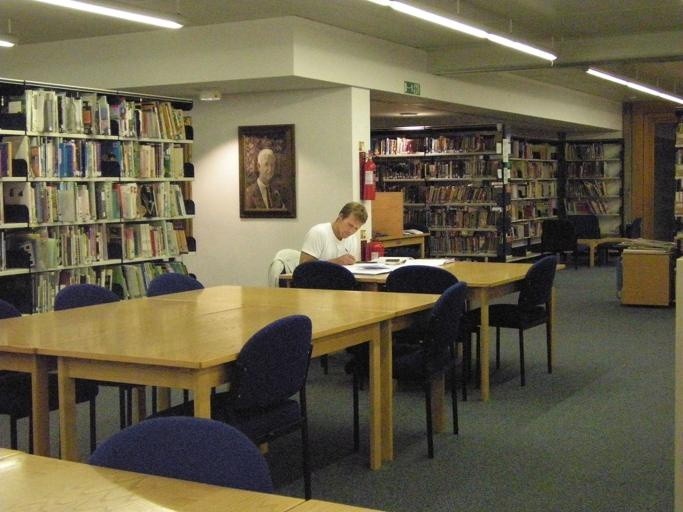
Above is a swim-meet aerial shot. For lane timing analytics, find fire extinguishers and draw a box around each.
[363,151,377,200]
[367,239,384,262]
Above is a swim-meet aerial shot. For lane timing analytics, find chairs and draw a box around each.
[143,271,200,296]
[268,249,308,289]
[222,313,315,491]
[382,265,456,299]
[467,254,559,388]
[0,299,99,461]
[74,415,280,494]
[290,259,355,293]
[56,281,125,432]
[396,281,471,457]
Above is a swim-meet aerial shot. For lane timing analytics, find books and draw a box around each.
[371,134,607,262]
[349,255,455,274]
[673,122,683,253]
[0,88,189,314]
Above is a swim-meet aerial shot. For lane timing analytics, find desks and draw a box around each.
[1,298,245,456]
[1,448,25,459]
[0,448,305,511]
[146,283,444,460]
[619,240,670,311]
[375,231,431,257]
[294,497,384,512]
[408,258,566,400]
[277,259,380,288]
[36,304,392,470]
[292,497,380,511]
[376,260,568,402]
[279,239,453,396]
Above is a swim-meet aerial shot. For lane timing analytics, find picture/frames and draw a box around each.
[238,124,295,218]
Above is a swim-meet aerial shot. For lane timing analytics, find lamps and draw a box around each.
[582,64,682,113]
[55,1,186,36]
[394,2,488,44]
[481,16,558,70]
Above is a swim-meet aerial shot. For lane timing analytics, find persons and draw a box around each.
[244,149,285,208]
[300,202,368,264]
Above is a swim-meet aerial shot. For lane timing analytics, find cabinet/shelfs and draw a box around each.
[0,79,194,311]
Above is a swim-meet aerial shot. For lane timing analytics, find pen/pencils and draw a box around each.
[345,248,350,255]
